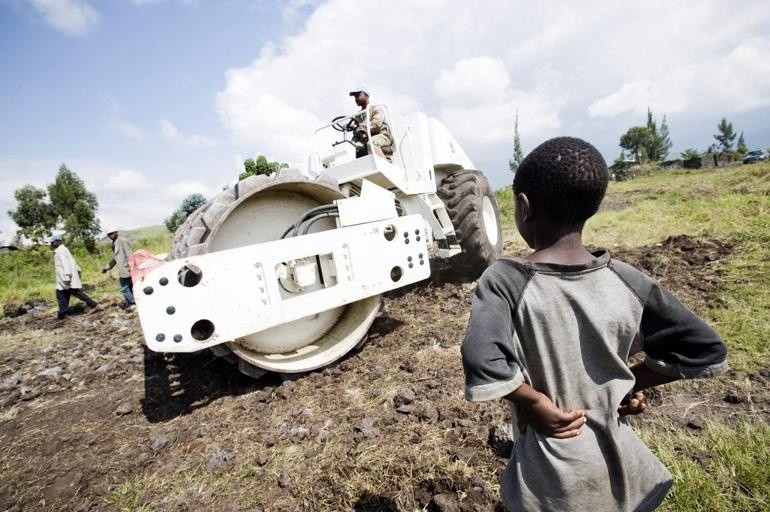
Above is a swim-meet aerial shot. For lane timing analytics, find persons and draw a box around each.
[461,136,727,512]
[46,236,100,323]
[348,86,392,162]
[102,226,135,309]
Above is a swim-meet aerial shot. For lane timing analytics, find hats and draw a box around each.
[107,226,116,234]
[350,88,370,97]
[48,236,60,242]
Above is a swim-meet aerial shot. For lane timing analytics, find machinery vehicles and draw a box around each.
[116,97,509,384]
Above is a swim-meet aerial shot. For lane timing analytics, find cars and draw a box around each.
[741,146,770,164]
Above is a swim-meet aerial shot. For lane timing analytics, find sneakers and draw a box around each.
[89,302,103,315]
